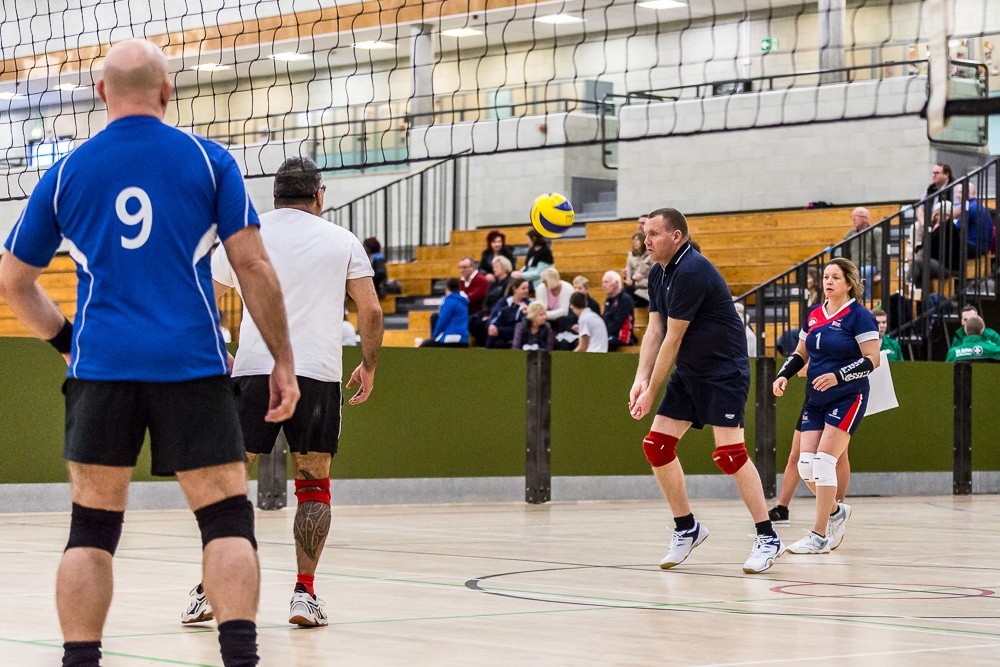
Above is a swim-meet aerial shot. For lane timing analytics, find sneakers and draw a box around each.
[742,529,786,574]
[289,590,328,627]
[786,528,831,555]
[829,503,852,550]
[660,519,710,569]
[181,582,216,624]
[767,506,790,524]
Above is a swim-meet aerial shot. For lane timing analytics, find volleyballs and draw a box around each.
[529,192,575,238]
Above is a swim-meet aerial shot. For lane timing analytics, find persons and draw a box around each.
[629,208,786,574]
[178,157,383,627]
[769,364,850,523]
[773,257,880,552]
[343,161,1000,364]
[0,37,301,667]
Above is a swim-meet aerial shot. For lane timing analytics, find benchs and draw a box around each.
[339,197,996,358]
